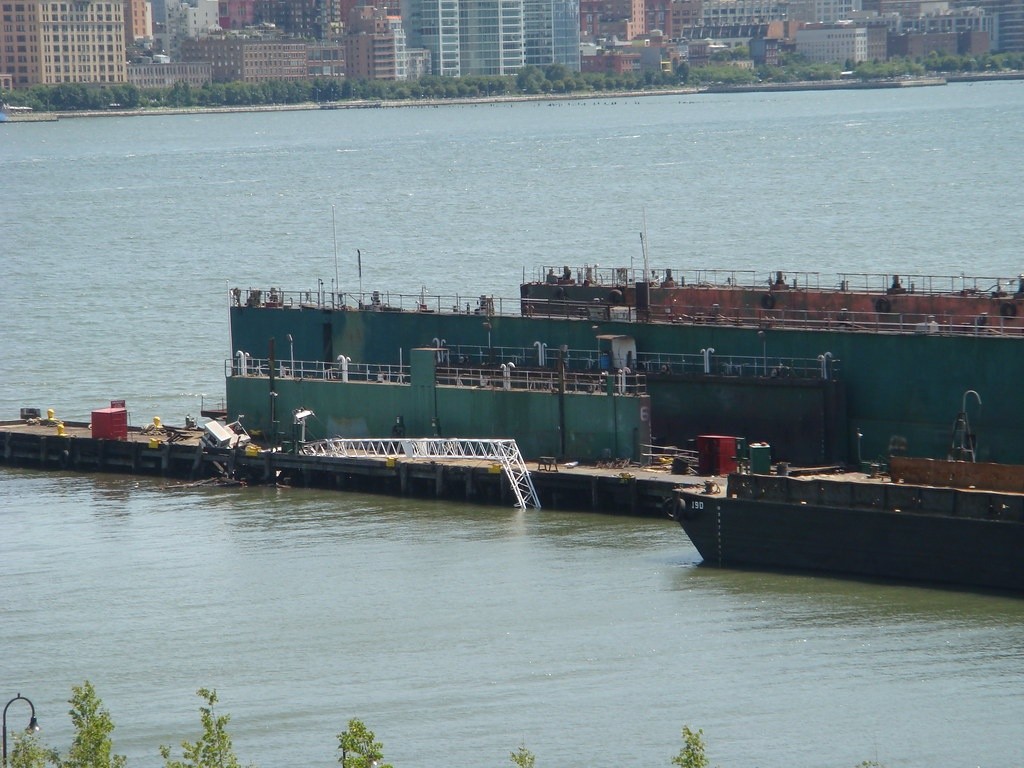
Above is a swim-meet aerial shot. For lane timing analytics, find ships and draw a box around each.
[224,220,1024,465]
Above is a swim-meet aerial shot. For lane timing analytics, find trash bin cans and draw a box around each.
[750,442,771,475]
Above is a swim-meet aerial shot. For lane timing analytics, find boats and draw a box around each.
[664,473,1023,596]
